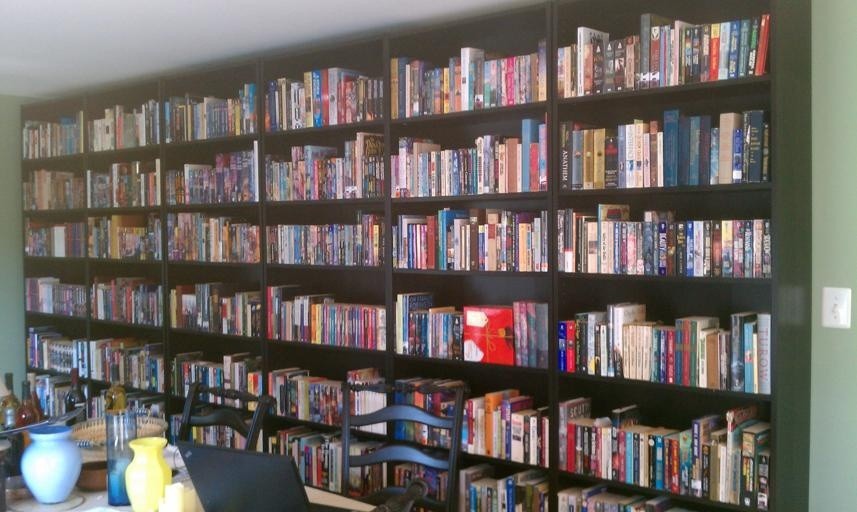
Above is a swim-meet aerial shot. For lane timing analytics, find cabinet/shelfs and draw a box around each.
[20,3,809,511]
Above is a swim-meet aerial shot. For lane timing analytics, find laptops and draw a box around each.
[176,440,355,512]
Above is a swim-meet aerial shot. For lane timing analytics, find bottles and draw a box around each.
[0,367,173,512]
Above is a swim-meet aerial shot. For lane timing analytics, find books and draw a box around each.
[265,66,387,506]
[556,14,774,512]
[23,81,263,452]
[390,37,549,510]
